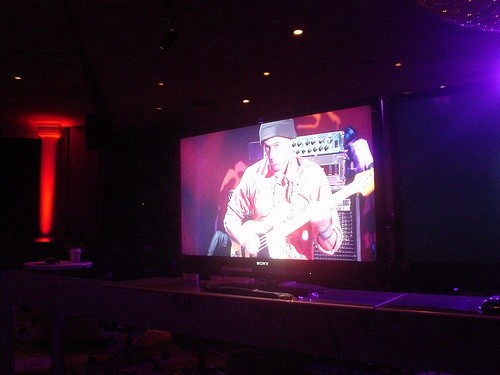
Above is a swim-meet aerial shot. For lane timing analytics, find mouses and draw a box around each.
[481,295,500,316]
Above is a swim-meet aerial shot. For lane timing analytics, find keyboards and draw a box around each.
[204,282,308,301]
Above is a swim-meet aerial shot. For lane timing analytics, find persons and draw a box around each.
[223,118,344,259]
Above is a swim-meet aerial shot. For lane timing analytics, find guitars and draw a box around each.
[229,168,375,258]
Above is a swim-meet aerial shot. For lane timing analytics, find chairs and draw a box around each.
[85,256,172,372]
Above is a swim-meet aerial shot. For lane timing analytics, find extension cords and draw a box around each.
[27,256,92,270]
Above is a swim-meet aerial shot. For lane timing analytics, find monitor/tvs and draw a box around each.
[0,136,39,254]
[169,96,390,276]
[389,80,498,267]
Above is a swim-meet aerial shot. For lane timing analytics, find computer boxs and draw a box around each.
[58,124,166,278]
